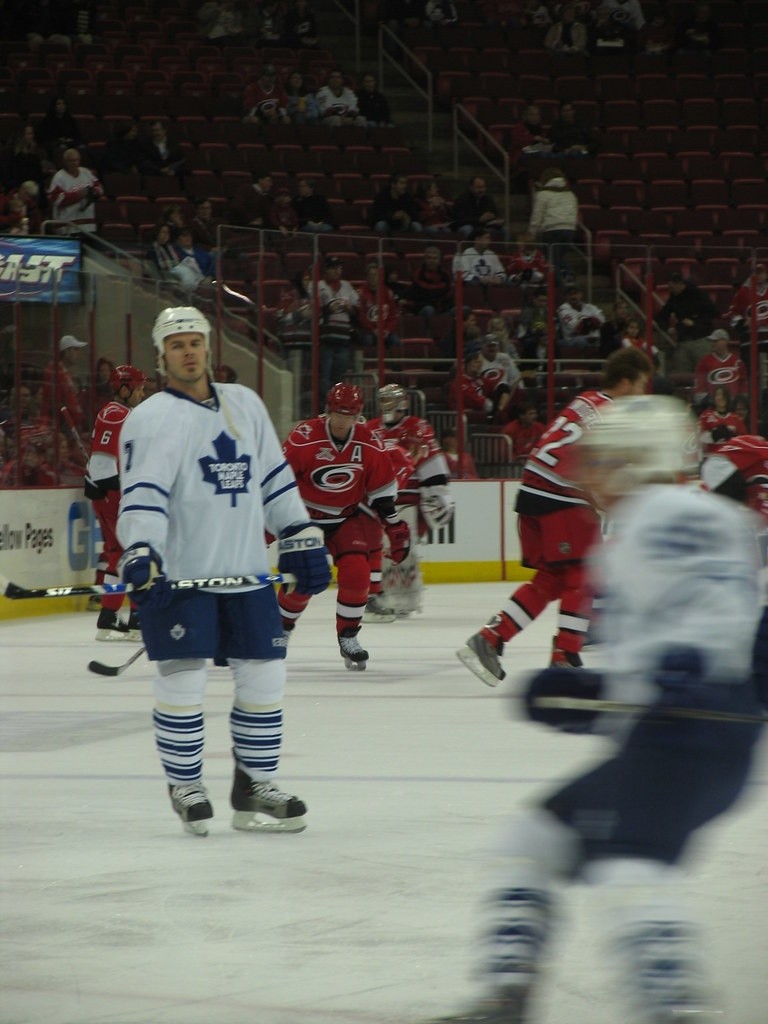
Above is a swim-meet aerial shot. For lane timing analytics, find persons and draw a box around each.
[455,343,656,688]
[114,304,333,837]
[84,365,146,643]
[698,431,767,518]
[426,390,768,1024]
[360,382,453,624]
[265,380,412,671]
[0,0,768,484]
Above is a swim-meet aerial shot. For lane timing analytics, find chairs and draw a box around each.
[0,0,768,427]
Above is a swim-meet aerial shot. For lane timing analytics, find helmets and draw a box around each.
[378,384,408,422]
[326,381,364,415]
[112,364,150,396]
[151,306,212,374]
[587,396,696,495]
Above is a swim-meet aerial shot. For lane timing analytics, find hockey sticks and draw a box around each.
[61,406,117,516]
[3,573,297,602]
[532,696,768,724]
[88,646,146,677]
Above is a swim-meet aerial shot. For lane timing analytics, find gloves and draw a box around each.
[115,546,172,610]
[275,524,333,596]
[384,519,413,563]
[421,494,458,530]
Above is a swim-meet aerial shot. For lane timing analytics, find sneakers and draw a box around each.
[281,623,295,660]
[229,766,308,834]
[170,779,213,836]
[362,598,396,623]
[338,625,369,671]
[95,609,143,642]
[456,633,507,687]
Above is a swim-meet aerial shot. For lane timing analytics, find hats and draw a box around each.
[484,338,500,346]
[59,335,88,352]
[706,328,729,341]
[326,256,345,267]
